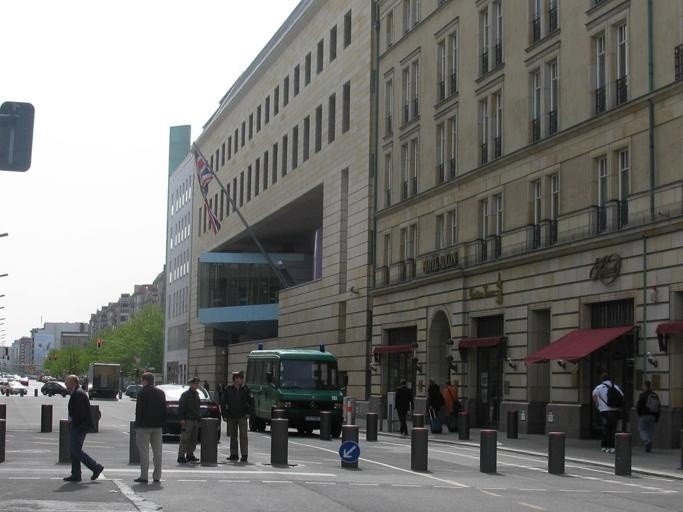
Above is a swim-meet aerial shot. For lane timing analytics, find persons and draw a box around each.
[637,381,661,451]
[177,377,201,463]
[60,375,104,482]
[217,380,224,404]
[441,380,458,430]
[592,372,625,453]
[203,379,210,391]
[394,378,415,436]
[134,372,167,483]
[425,379,443,428]
[221,370,255,462]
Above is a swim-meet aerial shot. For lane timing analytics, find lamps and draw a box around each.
[646,352,657,367]
[557,359,567,368]
[446,337,457,373]
[506,356,517,369]
[658,208,669,217]
[348,286,359,295]
[411,342,422,373]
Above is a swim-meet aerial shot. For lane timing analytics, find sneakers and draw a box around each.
[645,441,652,453]
[601,447,615,454]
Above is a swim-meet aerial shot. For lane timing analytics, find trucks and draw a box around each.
[82,362,120,400]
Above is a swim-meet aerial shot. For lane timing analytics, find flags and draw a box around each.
[192,149,229,236]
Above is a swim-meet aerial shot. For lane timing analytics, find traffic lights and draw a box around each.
[97,340,101,348]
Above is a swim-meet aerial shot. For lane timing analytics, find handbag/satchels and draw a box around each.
[452,399,461,411]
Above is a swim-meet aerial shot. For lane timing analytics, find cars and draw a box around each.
[156,383,222,444]
[30,375,65,382]
[0,372,29,396]
[41,381,70,397]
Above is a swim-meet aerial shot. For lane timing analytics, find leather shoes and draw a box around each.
[397,427,408,435]
[189,451,196,459]
[154,478,160,484]
[239,455,246,462]
[133,477,146,484]
[177,454,185,462]
[62,475,79,480]
[91,463,103,478]
[226,454,236,461]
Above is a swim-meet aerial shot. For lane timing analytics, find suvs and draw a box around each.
[126,385,144,399]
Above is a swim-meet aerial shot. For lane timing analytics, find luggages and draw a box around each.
[429,408,442,434]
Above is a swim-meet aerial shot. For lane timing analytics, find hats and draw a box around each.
[600,373,612,380]
[187,376,198,382]
[230,370,243,374]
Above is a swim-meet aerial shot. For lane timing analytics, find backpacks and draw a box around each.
[645,392,659,412]
[599,382,623,408]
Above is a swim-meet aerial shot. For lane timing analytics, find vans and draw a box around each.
[247,342,346,439]
